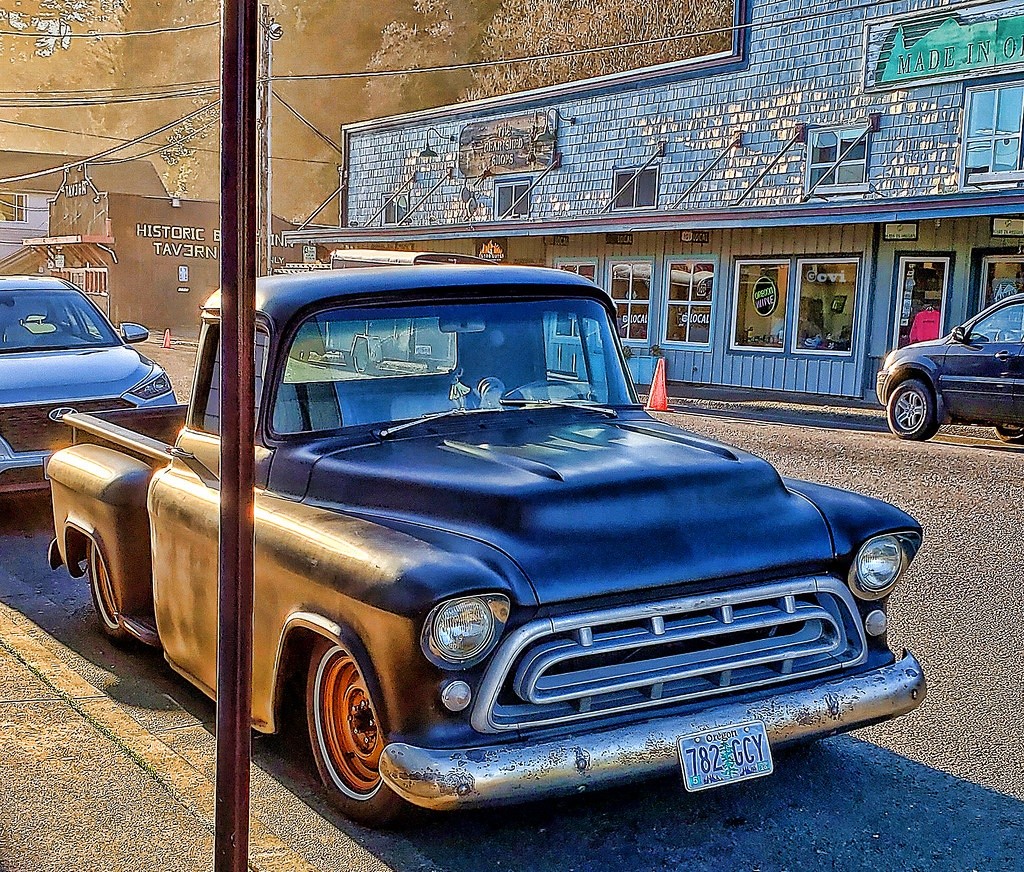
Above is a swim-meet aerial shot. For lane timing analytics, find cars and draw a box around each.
[875,293,1024,444]
[0,274,179,531]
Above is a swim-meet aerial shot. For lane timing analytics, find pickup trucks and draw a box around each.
[44,264,927,829]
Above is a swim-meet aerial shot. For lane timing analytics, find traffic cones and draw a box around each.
[644,358,674,413]
